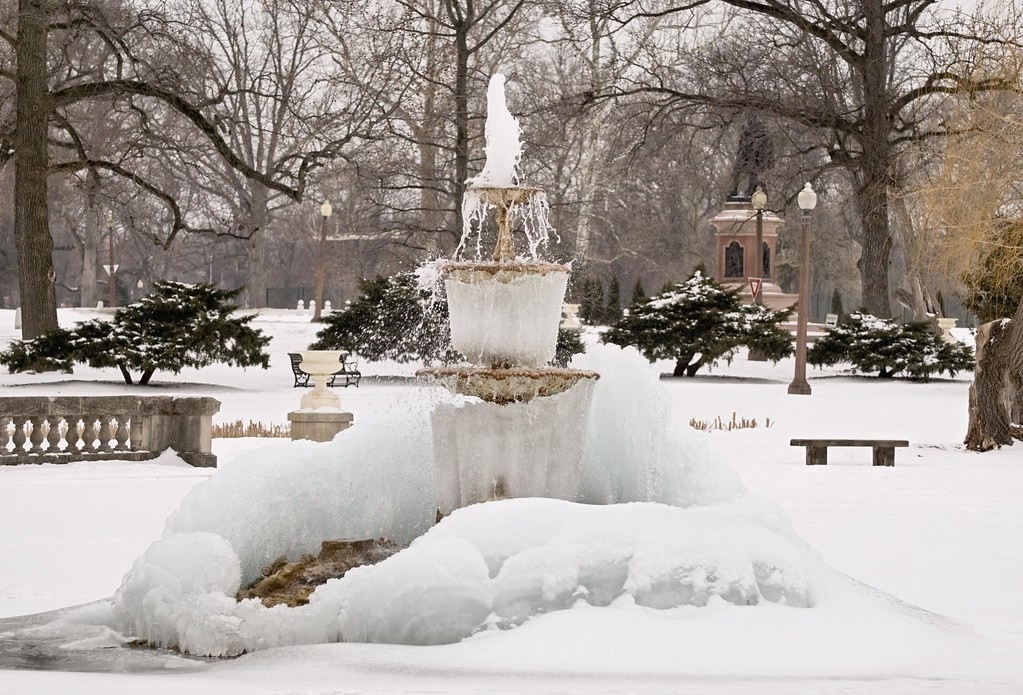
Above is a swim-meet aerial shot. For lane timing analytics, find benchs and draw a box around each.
[287,349,361,389]
[790,438,910,467]
